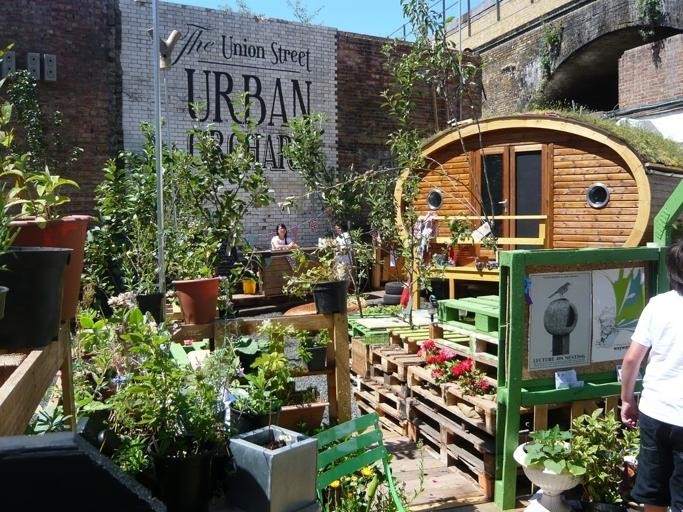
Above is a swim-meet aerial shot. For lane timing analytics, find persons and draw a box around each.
[619,239,683,512]
[331,221,353,295]
[269,224,299,250]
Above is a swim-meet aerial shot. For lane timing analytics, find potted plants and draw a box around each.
[350,337,390,378]
[0,39,356,512]
[513,407,641,512]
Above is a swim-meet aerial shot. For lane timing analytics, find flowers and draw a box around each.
[417,339,491,397]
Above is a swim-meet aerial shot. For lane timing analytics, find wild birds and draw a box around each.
[546,282,572,299]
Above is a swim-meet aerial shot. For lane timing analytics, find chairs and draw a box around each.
[311,412,407,512]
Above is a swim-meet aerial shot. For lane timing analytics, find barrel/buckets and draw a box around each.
[242,279,256,294]
[263,257,292,297]
[171,277,219,324]
[0,216,92,323]
